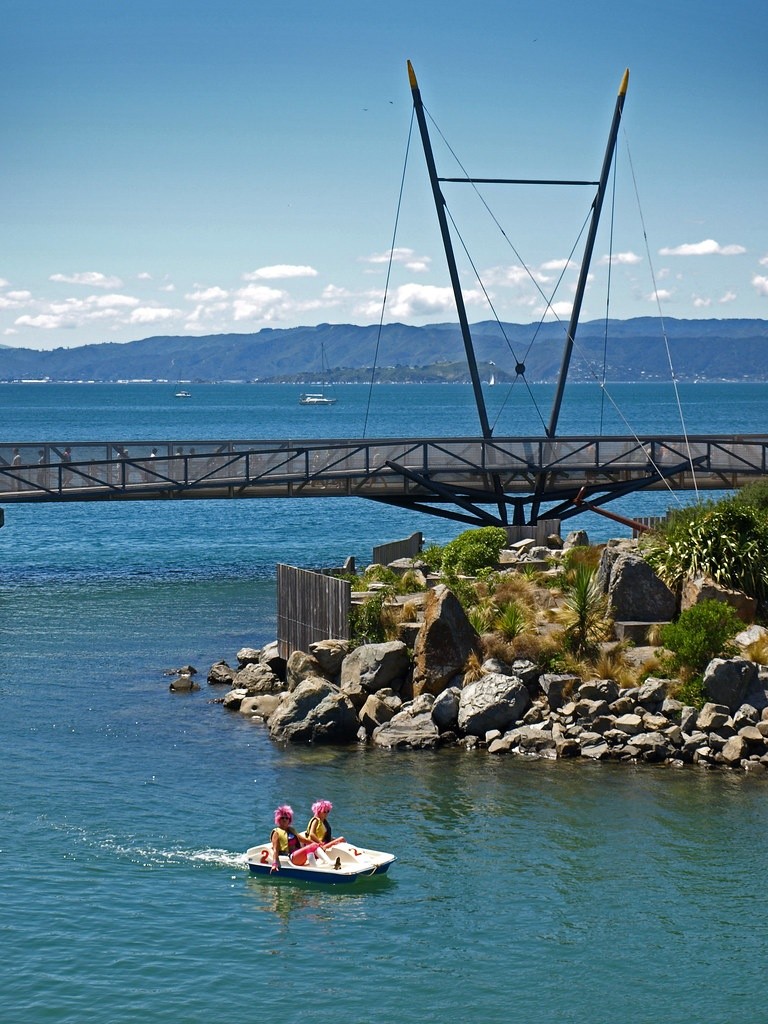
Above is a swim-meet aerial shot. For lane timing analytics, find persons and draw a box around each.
[270,805,340,874]
[307,800,332,846]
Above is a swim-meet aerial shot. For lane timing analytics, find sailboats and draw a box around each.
[298,343,340,406]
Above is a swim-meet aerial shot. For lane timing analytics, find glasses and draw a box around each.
[280,816,290,820]
[322,810,329,813]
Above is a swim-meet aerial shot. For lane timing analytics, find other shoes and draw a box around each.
[333,857,341,869]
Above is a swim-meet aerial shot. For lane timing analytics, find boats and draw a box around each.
[244,831,399,885]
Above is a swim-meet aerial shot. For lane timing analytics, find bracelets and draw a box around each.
[272,862,279,868]
